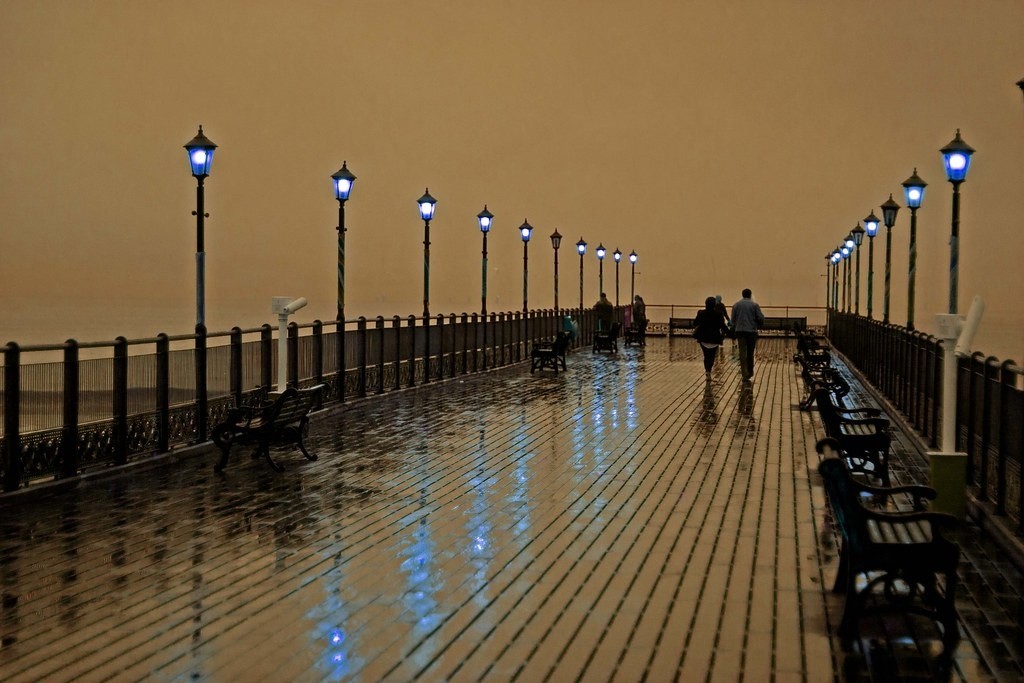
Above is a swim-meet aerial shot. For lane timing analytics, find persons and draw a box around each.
[693,288,765,380]
[593,293,613,335]
[632,295,645,322]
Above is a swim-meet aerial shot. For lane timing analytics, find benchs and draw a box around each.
[530,329,572,375]
[792,322,961,656]
[669,318,695,335]
[624,319,650,346]
[762,317,807,334]
[211,381,332,473]
[592,321,622,354]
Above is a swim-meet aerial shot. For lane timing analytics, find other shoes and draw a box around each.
[742,373,753,383]
[705,370,712,382]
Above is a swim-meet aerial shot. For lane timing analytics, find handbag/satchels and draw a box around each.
[692,324,705,340]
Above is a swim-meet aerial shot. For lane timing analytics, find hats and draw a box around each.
[716,295,721,301]
